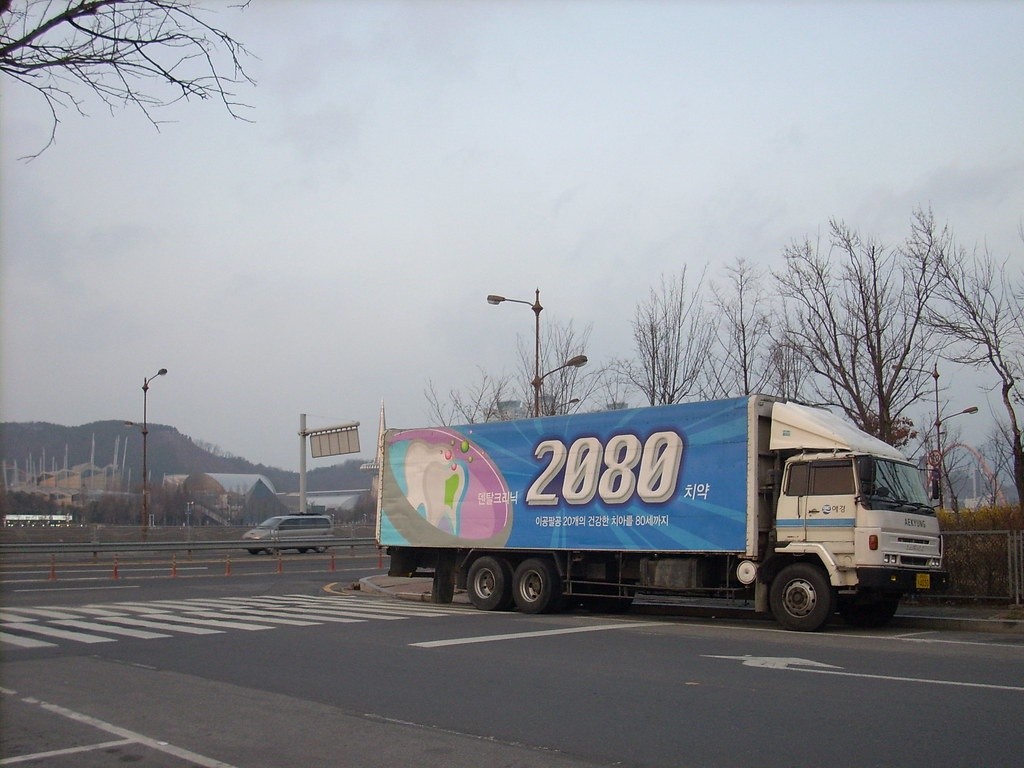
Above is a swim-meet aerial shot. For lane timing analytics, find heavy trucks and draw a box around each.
[373,392,948,631]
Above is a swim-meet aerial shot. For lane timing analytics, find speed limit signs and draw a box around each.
[927,450,942,466]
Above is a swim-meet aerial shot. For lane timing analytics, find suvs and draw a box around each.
[241,512,336,556]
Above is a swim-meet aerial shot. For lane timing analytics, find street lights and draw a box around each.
[891,362,978,511]
[122,368,168,542]
[486,287,588,417]
